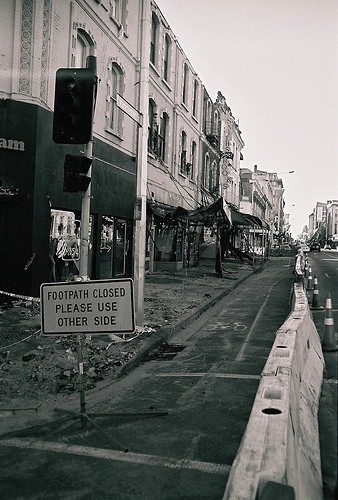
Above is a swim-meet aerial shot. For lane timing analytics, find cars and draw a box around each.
[324,245,331,250]
[291,239,321,254]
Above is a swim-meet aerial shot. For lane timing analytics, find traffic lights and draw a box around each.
[63,153,92,192]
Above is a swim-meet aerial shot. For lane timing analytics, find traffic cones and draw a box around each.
[319,292,338,352]
[305,264,314,290]
[305,253,309,263]
[309,275,324,310]
[304,260,308,278]
